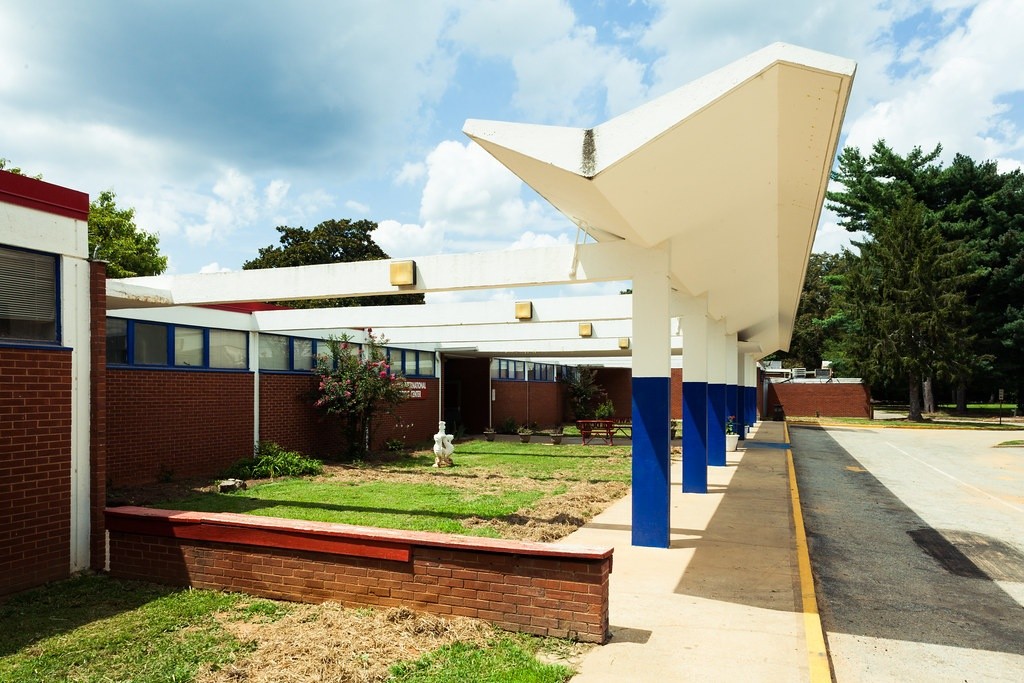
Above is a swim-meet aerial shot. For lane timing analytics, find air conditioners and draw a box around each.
[792,367,806,378]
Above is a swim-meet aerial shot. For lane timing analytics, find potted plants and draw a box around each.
[517,426,534,443]
[549,422,563,445]
[671,420,677,440]
[483,426,498,441]
[725,415,740,453]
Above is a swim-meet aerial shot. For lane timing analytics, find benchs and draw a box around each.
[606,425,632,438]
[582,428,617,434]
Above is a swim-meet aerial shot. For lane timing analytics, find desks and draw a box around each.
[576,420,615,445]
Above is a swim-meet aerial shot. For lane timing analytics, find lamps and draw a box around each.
[579,323,592,336]
[619,338,629,348]
[514,301,533,320]
[389,260,416,286]
